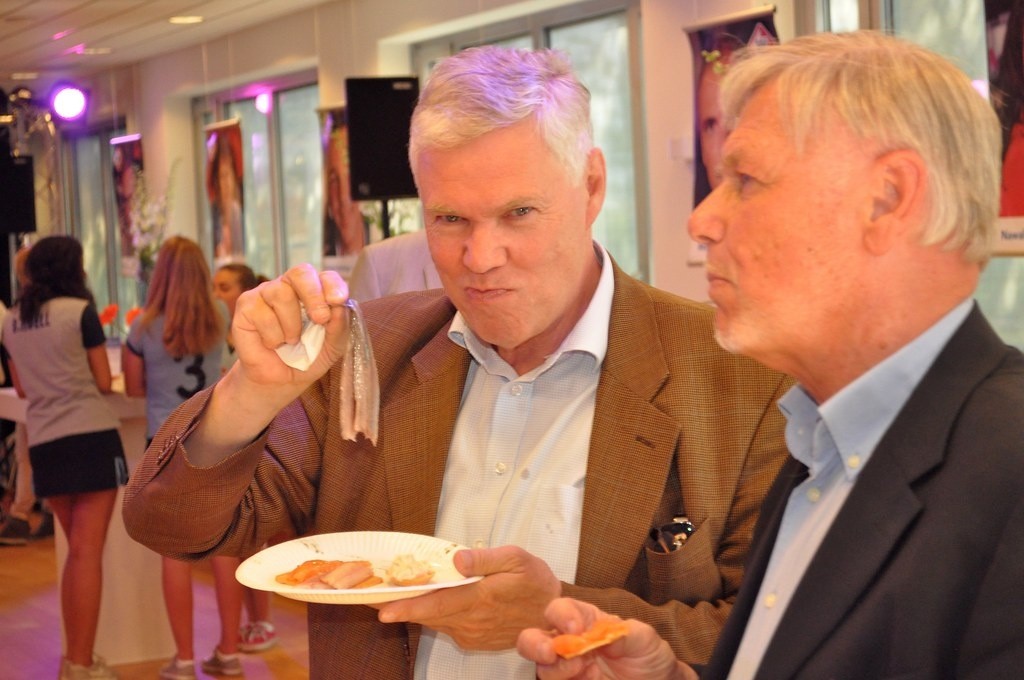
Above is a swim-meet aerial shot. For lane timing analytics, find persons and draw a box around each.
[120,48,794,679]
[0,234,133,679]
[126,236,247,677]
[516,27,1023,680]
[204,262,285,649]
[0,248,59,543]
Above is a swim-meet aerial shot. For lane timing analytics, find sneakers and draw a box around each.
[201,647,243,676]
[60,651,121,680]
[157,654,196,680]
[237,623,281,652]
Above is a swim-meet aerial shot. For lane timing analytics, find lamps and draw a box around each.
[46,81,91,134]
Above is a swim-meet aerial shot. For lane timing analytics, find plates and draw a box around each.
[233,531,484,606]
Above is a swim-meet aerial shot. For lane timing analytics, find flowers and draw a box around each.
[130,147,185,269]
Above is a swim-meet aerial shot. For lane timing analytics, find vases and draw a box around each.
[133,269,152,309]
